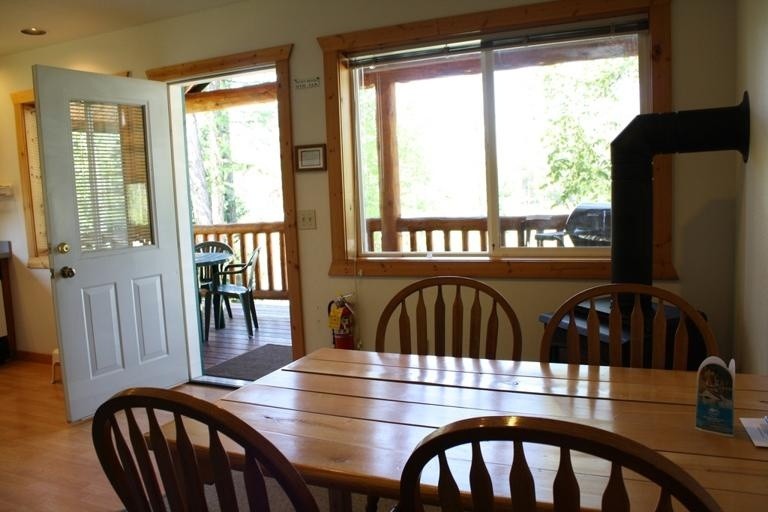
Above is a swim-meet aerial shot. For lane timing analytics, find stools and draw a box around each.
[50,349,61,384]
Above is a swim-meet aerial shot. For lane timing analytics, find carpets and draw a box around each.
[206,344,293,381]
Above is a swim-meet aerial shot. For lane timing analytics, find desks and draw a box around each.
[194,252,233,330]
[141,345,766,512]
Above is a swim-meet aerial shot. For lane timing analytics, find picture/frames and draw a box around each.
[293,144,327,171]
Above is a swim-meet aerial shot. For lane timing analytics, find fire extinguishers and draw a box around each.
[328,294,356,349]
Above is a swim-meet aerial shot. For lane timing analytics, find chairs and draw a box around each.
[399,416,724,512]
[366,276,522,512]
[195,242,236,318]
[205,246,266,343]
[540,284,718,369]
[92,387,321,512]
